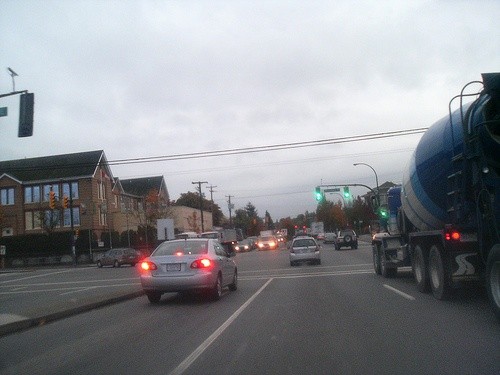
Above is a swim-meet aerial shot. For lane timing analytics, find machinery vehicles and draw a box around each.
[370,72,499,317]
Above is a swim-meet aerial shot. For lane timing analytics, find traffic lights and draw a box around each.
[380,211,388,219]
[316,186,321,201]
[343,186,350,199]
[49,191,55,209]
[62,195,67,209]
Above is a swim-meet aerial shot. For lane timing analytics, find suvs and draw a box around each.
[334,229,358,250]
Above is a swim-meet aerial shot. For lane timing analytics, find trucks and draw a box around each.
[260,231,273,237]
[202,228,237,257]
[174,232,199,238]
[310,221,324,238]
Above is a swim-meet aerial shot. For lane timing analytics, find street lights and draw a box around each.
[353,163,382,232]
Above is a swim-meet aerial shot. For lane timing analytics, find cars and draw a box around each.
[96,247,142,267]
[286,236,320,265]
[137,238,238,299]
[243,236,258,249]
[235,241,251,252]
[257,235,277,251]
[324,233,337,243]
[316,233,326,239]
